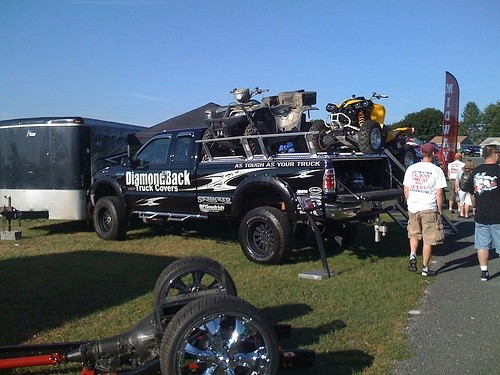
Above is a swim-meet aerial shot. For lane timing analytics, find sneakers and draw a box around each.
[421,266,430,276]
[481,271,490,280]
[408,255,417,272]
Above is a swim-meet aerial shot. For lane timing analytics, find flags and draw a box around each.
[441,71,460,164]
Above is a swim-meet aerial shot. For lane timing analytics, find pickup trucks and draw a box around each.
[84,126,405,264]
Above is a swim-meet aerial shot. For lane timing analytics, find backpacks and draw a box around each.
[459,170,472,191]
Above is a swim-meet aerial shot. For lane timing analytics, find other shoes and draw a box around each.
[460,216,464,218]
[472,213,476,216]
[465,217,469,219]
[449,210,456,213]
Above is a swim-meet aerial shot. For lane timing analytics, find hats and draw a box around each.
[455,153,462,158]
[421,143,435,152]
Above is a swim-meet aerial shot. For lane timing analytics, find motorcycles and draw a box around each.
[306,91,388,154]
[380,122,419,169]
[201,86,319,159]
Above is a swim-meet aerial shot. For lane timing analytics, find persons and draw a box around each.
[466,144,500,282]
[455,159,475,219]
[401,142,447,276]
[450,151,466,218]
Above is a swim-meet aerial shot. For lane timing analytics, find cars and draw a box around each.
[458,145,481,157]
